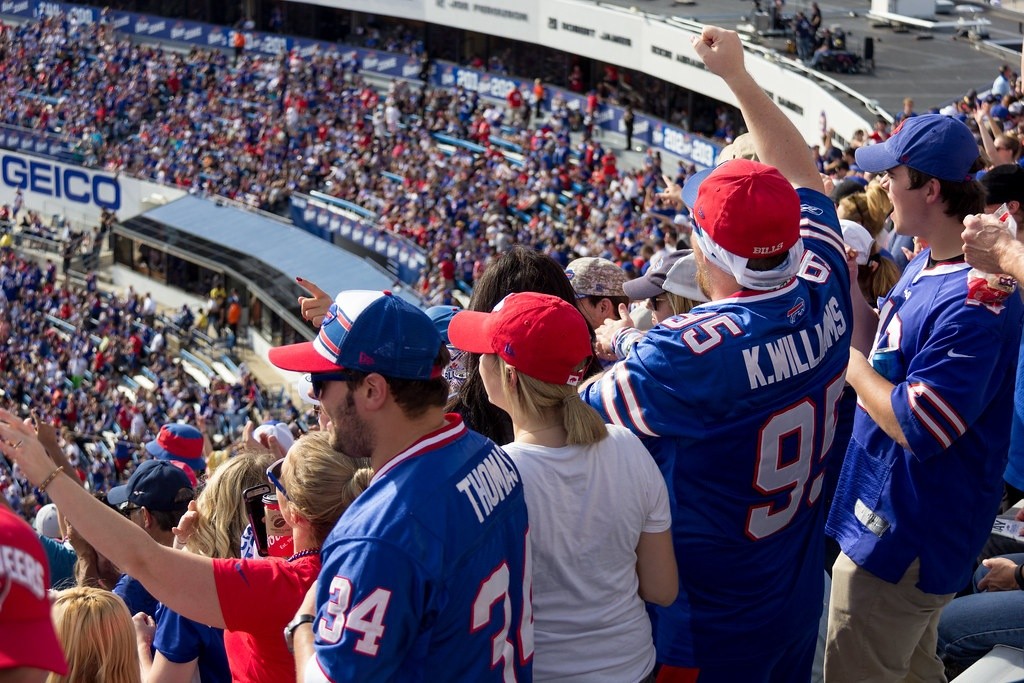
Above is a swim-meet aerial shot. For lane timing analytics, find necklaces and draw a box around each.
[287,550,320,563]
[516,421,564,438]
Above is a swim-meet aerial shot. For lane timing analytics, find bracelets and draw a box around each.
[38,465,64,492]
[176,540,186,544]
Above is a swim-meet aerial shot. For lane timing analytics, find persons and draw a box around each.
[575,27,854,683]
[0,0,1024,659]
[824,111,1024,683]
[962,213,1024,492]
[49,587,141,683]
[130,452,275,683]
[446,291,680,683]
[268,290,533,683]
[0,407,374,683]
[936,553,1024,683]
[0,506,68,683]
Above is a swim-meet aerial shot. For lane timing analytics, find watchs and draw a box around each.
[283,614,316,654]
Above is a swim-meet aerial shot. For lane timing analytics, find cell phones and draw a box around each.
[243,484,272,556]
[295,419,308,433]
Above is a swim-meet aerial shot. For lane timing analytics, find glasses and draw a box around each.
[265,458,291,500]
[650,296,669,311]
[995,146,1009,151]
[310,371,362,397]
[117,501,143,517]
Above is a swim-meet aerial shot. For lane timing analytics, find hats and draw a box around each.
[146,423,206,471]
[680,159,802,258]
[564,255,629,296]
[425,305,462,344]
[35,503,64,538]
[108,460,199,514]
[855,114,981,181]
[839,220,876,265]
[268,290,446,379]
[661,252,712,303]
[447,292,594,385]
[254,424,294,453]
[623,248,692,299]
[982,164,1024,204]
